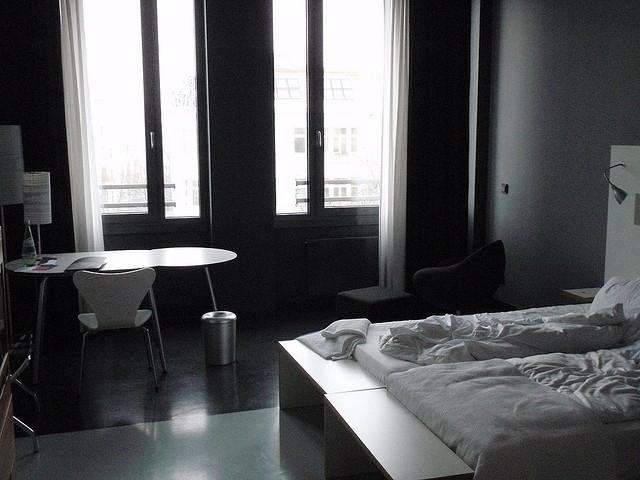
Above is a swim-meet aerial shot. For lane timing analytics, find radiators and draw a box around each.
[302,235,380,298]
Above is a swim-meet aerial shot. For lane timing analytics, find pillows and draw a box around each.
[589,277,640,319]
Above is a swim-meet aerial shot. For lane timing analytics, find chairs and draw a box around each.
[409,239,509,314]
[72,267,160,392]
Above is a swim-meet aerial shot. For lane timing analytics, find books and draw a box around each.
[64,256,107,271]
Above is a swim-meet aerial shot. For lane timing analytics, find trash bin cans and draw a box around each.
[202,311,237,365]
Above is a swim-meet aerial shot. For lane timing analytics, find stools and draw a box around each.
[321,390,475,480]
[336,279,409,318]
[277,335,384,412]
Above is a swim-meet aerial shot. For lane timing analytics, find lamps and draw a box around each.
[21,172,52,261]
[603,162,627,207]
[0,125,25,359]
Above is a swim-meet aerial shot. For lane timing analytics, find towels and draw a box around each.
[295,317,371,360]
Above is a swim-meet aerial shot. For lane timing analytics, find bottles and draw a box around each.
[21,219,36,266]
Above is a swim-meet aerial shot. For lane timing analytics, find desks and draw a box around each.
[4,246,239,375]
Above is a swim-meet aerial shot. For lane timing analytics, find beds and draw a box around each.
[333,144,640,480]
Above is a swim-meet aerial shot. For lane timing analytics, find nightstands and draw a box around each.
[559,284,605,304]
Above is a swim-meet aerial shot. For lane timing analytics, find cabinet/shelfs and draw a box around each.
[0,354,17,480]
